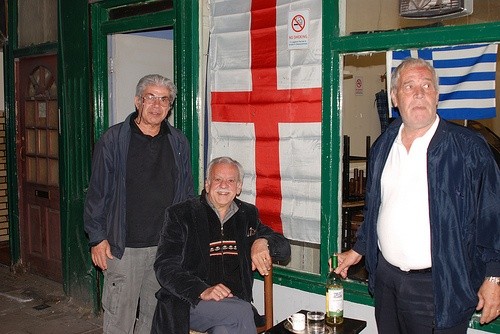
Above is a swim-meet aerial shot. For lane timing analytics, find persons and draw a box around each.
[329,58,500,334]
[152,158,291,333]
[83,74,193,334]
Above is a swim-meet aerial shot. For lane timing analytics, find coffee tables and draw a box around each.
[265,310,367,334]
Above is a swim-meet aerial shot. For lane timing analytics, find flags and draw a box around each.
[387,44,498,118]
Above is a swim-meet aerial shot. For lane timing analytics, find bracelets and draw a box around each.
[486,277,500,285]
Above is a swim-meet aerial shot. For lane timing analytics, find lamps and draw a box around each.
[398,0,474,21]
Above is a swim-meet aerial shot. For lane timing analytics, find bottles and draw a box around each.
[325,253,343,324]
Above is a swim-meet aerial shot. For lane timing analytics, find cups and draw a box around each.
[307,311,325,334]
[287,314,305,331]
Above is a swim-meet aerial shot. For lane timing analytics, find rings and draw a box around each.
[263,258,266,261]
[219,294,222,297]
[497,310,500,313]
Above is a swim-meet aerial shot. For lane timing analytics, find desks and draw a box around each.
[341,135,371,250]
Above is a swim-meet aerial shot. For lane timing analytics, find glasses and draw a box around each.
[141,94,172,105]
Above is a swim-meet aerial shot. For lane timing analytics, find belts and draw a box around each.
[378,251,432,276]
